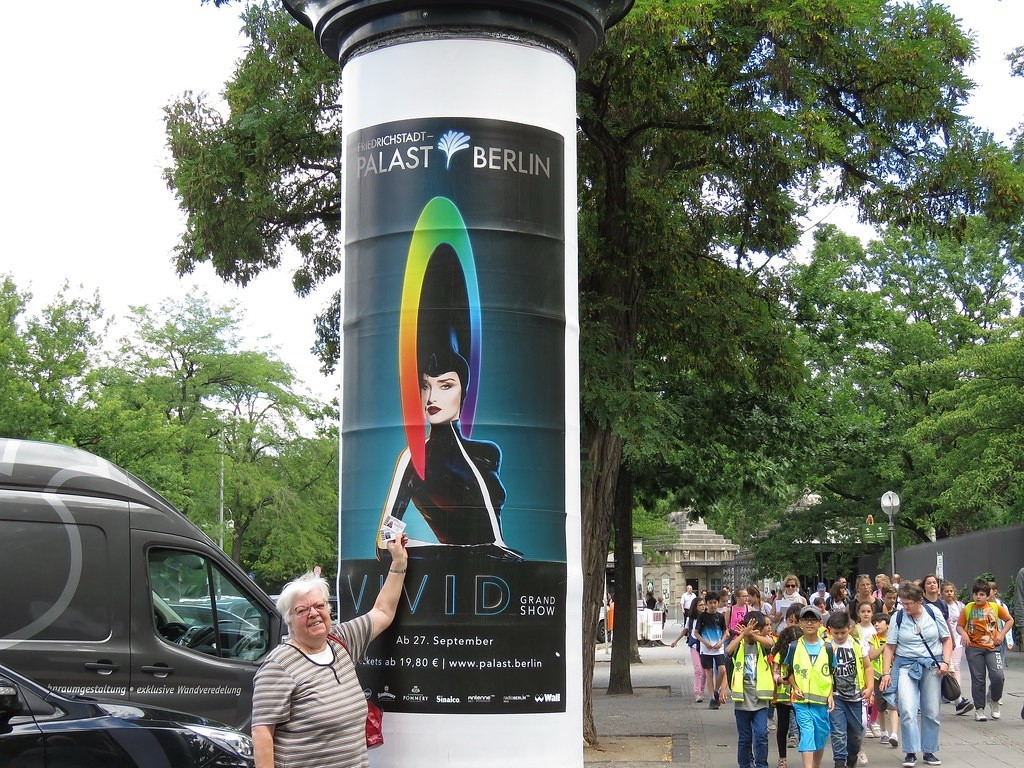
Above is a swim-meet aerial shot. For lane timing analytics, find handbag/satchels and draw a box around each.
[366,700,384,750]
[942,673,960,701]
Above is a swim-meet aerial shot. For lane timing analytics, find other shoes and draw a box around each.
[788,737,799,748]
[709,700,720,709]
[871,724,881,737]
[777,760,788,768]
[697,693,706,702]
[767,718,777,730]
[865,728,874,738]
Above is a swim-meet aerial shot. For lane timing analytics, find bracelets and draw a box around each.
[871,691,876,696]
[942,660,950,668]
[388,568,407,574]
[881,673,891,676]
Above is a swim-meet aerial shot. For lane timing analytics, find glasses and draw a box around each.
[786,584,796,589]
[899,600,918,606]
[801,618,818,624]
[295,601,326,615]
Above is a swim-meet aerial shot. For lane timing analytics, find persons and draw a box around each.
[607,592,614,648]
[670,566,1024,768]
[783,605,838,768]
[725,610,776,768]
[956,578,1014,722]
[725,611,774,768]
[694,591,728,711]
[249,532,410,768]
[644,592,668,631]
[878,579,954,767]
[826,610,875,767]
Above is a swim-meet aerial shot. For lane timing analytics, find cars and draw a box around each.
[167,595,337,632]
[0,666,256,768]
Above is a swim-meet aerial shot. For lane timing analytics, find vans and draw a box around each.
[0,437,287,737]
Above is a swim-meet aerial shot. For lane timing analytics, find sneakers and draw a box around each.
[857,751,868,767]
[975,708,987,721]
[888,737,899,747]
[990,701,1001,719]
[879,736,889,744]
[903,754,917,766]
[923,753,941,766]
[956,700,974,715]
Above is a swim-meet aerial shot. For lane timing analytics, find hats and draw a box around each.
[817,583,826,592]
[800,605,823,620]
